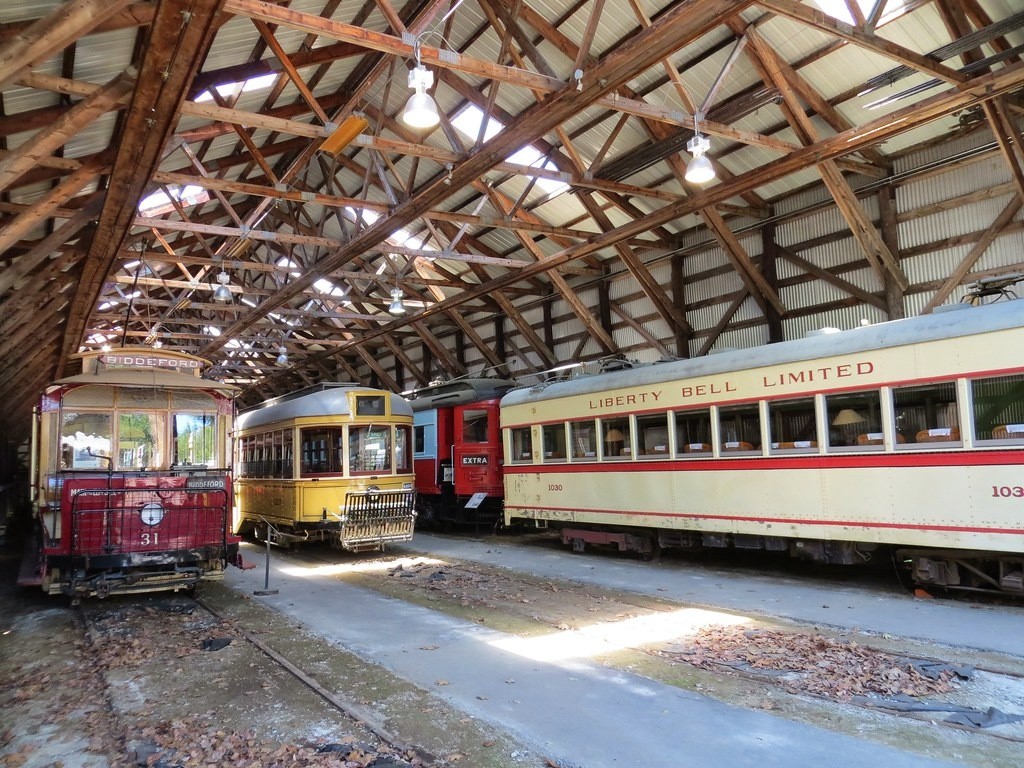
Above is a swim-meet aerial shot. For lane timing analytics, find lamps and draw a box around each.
[832,410,866,446]
[604,429,626,456]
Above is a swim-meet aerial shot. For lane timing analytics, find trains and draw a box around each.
[397,274,1024,597]
[30,344,256,610]
[227,382,417,555]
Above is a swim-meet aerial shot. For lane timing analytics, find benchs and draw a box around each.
[756,441,817,450]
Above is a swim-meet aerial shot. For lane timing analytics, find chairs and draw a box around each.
[620,448,644,455]
[684,443,712,453]
[579,452,595,457]
[721,442,753,451]
[915,428,959,443]
[991,424,1024,439]
[518,452,532,460]
[648,445,668,454]
[857,432,905,445]
[544,451,562,459]
